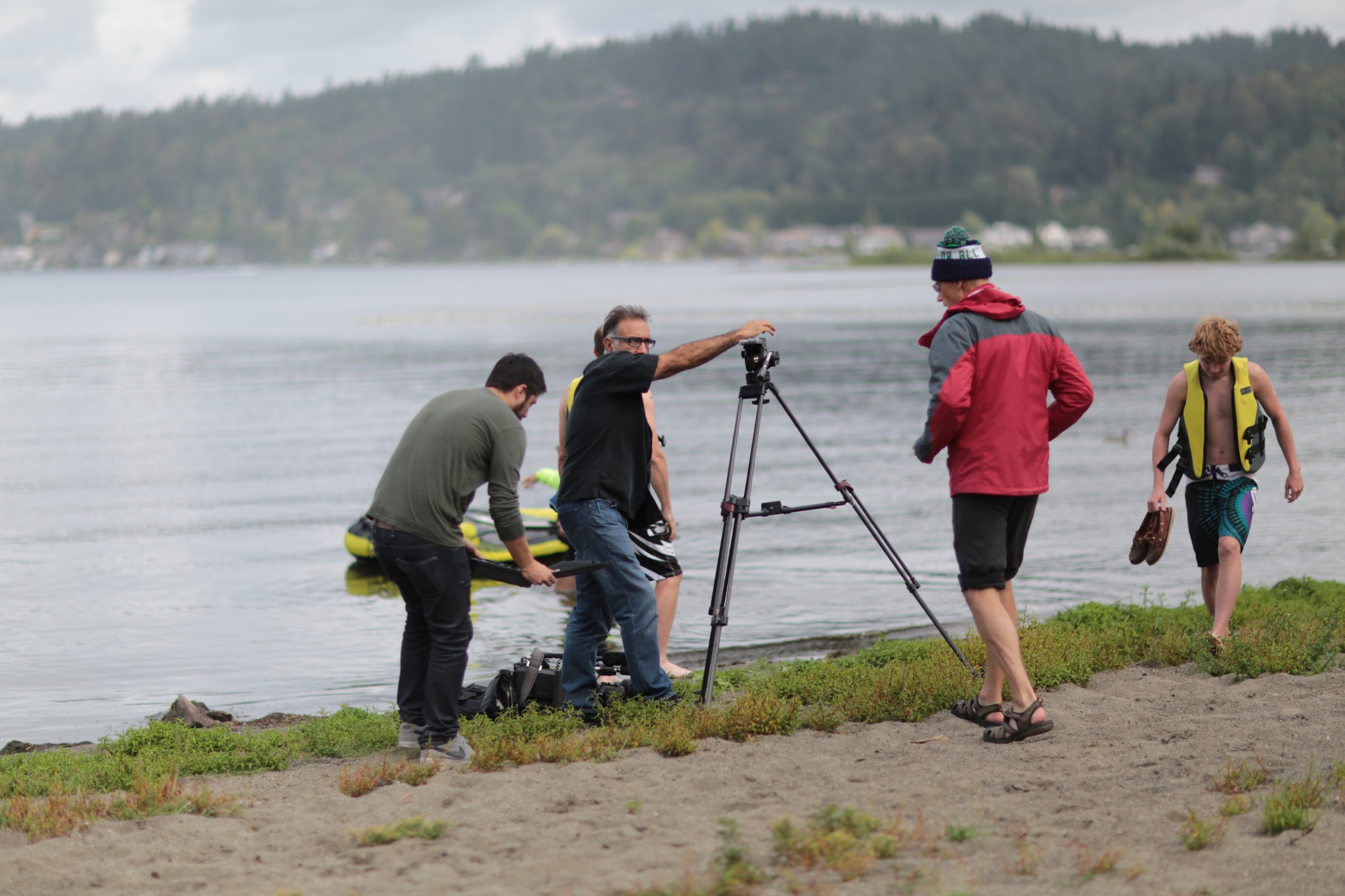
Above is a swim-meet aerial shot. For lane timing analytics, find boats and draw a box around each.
[346,505,568,569]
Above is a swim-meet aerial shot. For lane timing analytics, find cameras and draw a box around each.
[740,337,780,385]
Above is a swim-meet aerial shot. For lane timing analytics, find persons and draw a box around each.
[366,352,556,765]
[913,227,1097,744]
[523,305,778,721]
[1148,316,1305,653]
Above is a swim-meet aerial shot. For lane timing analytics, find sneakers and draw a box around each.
[398,722,429,748]
[420,731,476,765]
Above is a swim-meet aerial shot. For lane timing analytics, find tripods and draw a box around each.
[701,382,977,708]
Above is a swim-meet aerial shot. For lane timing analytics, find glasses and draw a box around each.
[932,281,940,292]
[609,337,656,349]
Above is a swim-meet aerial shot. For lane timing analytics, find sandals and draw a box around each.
[983,697,1053,744]
[951,695,1004,728]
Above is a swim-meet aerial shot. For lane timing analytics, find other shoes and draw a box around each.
[1145,507,1175,566]
[663,690,706,711]
[1128,512,1155,565]
[564,712,602,728]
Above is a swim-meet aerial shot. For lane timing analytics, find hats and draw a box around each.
[931,226,992,282]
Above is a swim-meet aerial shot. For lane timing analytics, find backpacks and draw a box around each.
[458,683,497,721]
